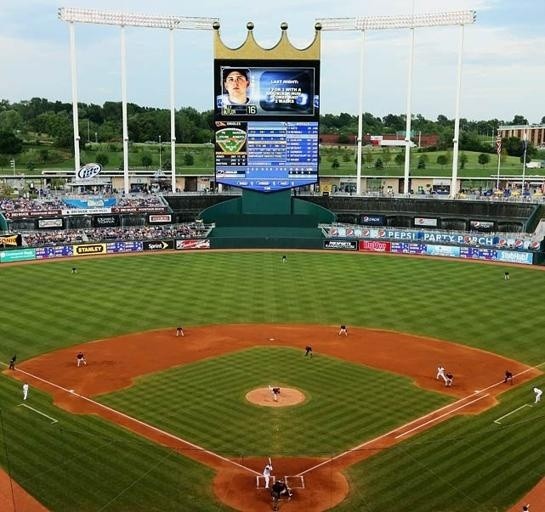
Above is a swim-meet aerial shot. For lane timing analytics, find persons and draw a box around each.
[8,353,17,372]
[76,351,87,368]
[0,179,220,250]
[223,68,251,106]
[443,370,453,388]
[70,265,77,275]
[532,386,544,403]
[270,480,284,511]
[175,327,185,337]
[263,462,274,490]
[503,268,510,280]
[436,364,447,382]
[338,326,349,337]
[22,380,29,400]
[328,184,545,241]
[303,344,313,360]
[503,367,514,385]
[267,384,280,402]
[282,255,288,264]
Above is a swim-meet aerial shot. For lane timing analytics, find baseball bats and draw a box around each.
[268,456,271,465]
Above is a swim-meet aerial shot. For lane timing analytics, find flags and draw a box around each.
[522,127,528,153]
[496,135,502,155]
